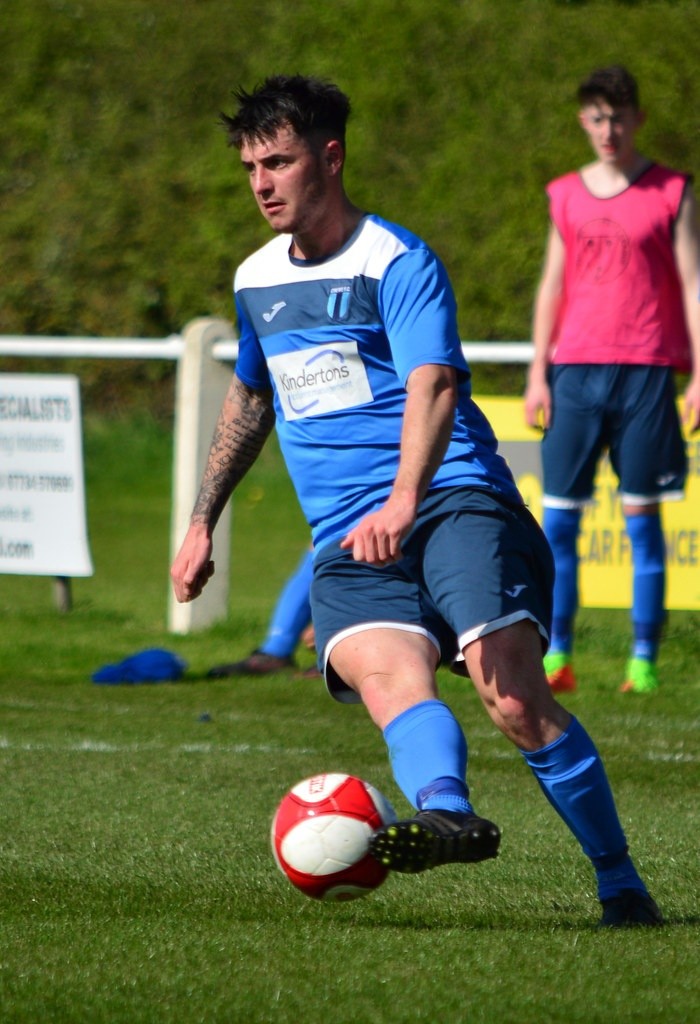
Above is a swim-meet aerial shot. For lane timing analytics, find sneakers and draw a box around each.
[368,809,502,874]
[599,890,662,929]
[546,658,578,692]
[623,656,655,695]
[204,648,295,677]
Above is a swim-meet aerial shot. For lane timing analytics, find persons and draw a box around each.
[522,63,700,698]
[167,70,666,933]
[236,540,317,675]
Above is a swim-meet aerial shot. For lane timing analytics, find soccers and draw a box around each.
[270,772,399,903]
